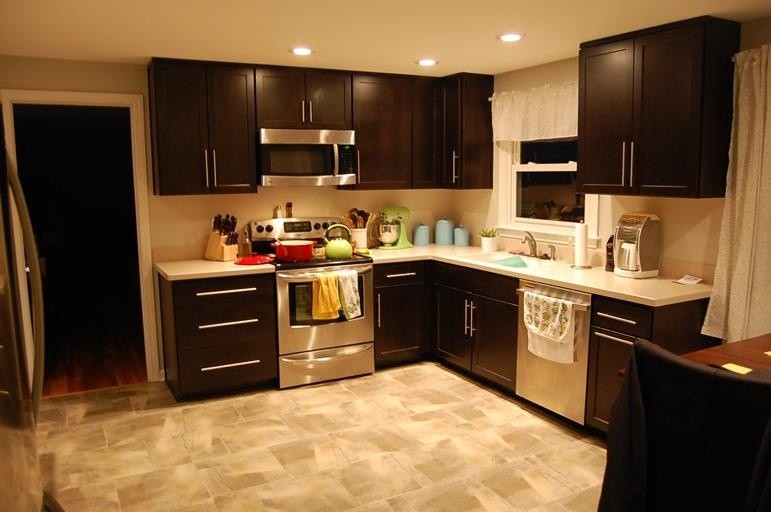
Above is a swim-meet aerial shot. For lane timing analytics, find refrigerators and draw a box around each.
[0,104,56,512]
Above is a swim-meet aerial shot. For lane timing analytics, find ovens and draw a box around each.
[278,260,379,396]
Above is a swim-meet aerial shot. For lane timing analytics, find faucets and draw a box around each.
[522,235,535,255]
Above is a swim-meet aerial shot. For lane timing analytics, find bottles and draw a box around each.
[286,202,293,218]
[238,226,252,258]
[276,205,282,218]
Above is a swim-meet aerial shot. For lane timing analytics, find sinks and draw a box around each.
[491,254,568,276]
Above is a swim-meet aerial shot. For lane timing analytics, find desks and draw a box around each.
[680,333,771,382]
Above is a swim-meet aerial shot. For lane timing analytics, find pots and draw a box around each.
[271,239,316,261]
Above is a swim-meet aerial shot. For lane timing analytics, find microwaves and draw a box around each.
[256,128,358,188]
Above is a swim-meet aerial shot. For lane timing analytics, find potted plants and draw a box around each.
[478,227,498,253]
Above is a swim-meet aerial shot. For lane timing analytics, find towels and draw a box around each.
[312,272,341,321]
[524,291,575,364]
[338,270,362,319]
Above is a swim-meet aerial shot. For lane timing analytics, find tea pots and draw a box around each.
[321,224,353,258]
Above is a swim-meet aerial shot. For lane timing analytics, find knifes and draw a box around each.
[214,213,239,246]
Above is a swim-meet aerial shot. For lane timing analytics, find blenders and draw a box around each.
[371,206,413,249]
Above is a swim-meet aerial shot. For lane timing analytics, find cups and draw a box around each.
[434,217,454,246]
[454,226,469,247]
[414,223,432,245]
[350,229,368,248]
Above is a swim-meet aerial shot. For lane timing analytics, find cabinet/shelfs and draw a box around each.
[158,272,278,403]
[575,16,742,199]
[439,72,494,189]
[373,261,434,362]
[337,71,439,190]
[255,63,352,129]
[585,294,722,431]
[147,57,257,196]
[430,261,519,391]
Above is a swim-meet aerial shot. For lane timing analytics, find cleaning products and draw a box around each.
[359,216,364,228]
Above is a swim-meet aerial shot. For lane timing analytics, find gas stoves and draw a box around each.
[261,250,374,270]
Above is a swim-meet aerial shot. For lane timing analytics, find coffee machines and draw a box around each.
[613,212,663,278]
